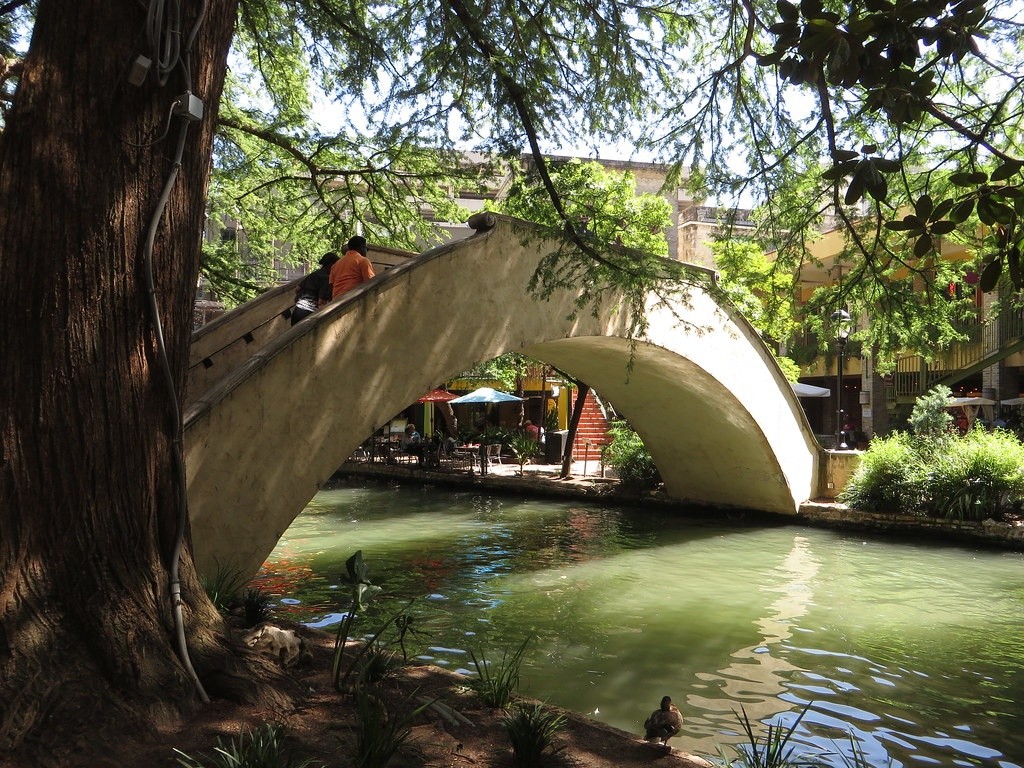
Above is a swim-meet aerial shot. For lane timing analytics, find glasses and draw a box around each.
[363,245,370,251]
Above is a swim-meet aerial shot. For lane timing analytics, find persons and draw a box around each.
[328,235,377,301]
[835,413,860,447]
[500,419,546,446]
[403,423,426,466]
[991,408,1024,442]
[292,252,341,327]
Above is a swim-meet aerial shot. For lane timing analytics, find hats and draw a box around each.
[523,420,531,425]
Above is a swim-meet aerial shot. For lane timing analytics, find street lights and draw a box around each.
[832,309,852,449]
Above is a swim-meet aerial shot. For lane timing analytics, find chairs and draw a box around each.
[348,437,421,468]
[443,439,502,475]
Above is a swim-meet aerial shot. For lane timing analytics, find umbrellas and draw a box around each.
[447,387,525,444]
[793,382,831,398]
[418,388,462,442]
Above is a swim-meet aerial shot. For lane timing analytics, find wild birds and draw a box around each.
[641,695,686,760]
